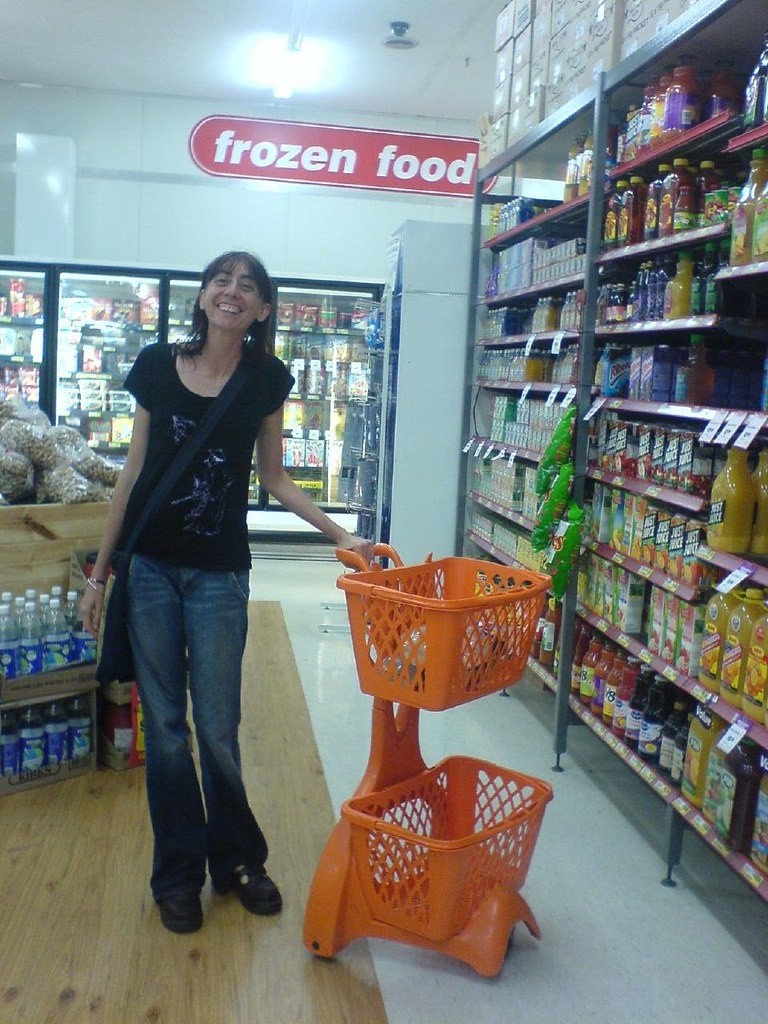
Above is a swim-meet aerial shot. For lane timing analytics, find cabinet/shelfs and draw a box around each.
[453,0,768,903]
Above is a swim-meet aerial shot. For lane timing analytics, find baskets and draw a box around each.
[336,557,554,711]
[340,755,554,943]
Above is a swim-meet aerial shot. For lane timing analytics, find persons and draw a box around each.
[79,249,376,935]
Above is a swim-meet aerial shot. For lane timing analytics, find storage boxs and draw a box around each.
[471,237,767,679]
[479,0,699,168]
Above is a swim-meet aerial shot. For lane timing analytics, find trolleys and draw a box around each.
[301,539,555,980]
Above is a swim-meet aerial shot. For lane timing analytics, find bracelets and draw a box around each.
[86,578,106,591]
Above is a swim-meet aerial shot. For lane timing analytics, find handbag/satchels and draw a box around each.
[93,555,135,686]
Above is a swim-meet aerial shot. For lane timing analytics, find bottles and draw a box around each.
[464,64,768,884]
[0,695,91,778]
[0,586,98,680]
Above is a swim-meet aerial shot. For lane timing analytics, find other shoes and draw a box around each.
[208,861,282,916]
[154,893,204,934]
